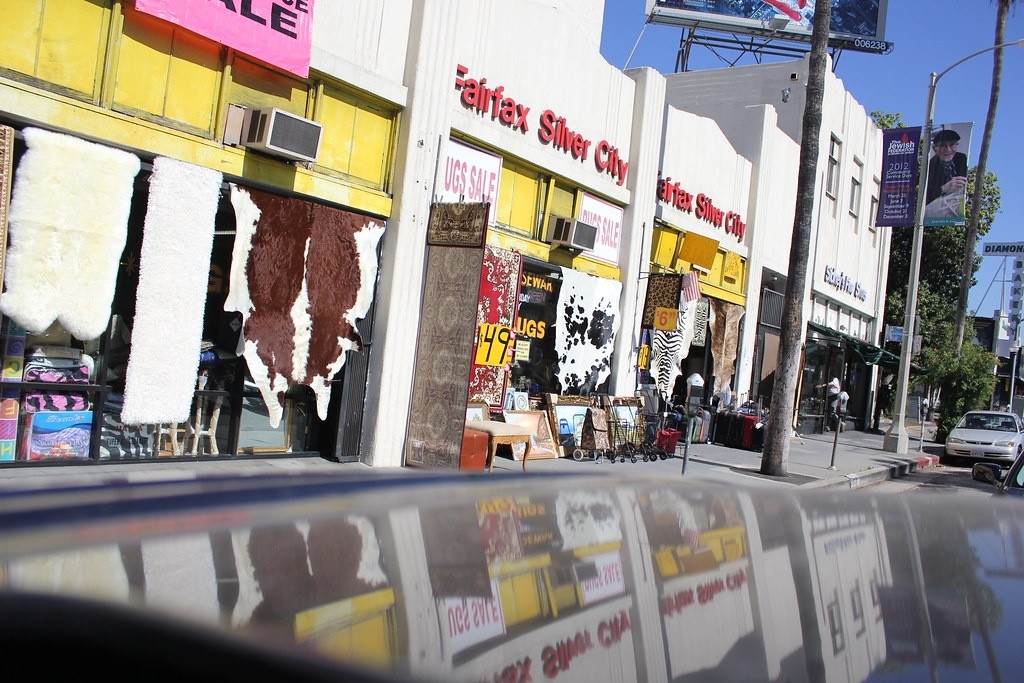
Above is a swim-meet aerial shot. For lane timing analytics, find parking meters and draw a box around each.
[827,391,849,471]
[681,372,705,476]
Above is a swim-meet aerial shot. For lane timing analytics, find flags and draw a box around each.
[683,272,701,302]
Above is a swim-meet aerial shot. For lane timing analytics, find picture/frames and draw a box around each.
[466,404,490,420]
[603,395,646,450]
[546,393,595,458]
[502,410,559,462]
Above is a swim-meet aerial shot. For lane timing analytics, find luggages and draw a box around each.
[659,391,771,452]
[572,392,610,464]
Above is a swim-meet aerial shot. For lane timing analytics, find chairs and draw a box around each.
[981,421,987,428]
[997,421,1014,428]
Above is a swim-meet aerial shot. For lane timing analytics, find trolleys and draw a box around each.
[572,389,675,462]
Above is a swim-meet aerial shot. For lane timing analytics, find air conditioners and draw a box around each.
[546,215,599,253]
[240,107,324,163]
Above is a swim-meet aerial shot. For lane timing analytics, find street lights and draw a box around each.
[1006,340,1019,412]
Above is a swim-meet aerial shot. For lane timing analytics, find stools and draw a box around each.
[465,420,533,474]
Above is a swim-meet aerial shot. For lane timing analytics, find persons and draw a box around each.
[815,369,846,432]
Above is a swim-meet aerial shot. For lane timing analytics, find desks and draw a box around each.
[169,388,229,456]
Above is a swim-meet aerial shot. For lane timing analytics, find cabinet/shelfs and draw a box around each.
[0,313,247,469]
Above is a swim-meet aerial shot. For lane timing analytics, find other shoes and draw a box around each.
[840,422,846,432]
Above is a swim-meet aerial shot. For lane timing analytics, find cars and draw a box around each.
[945,411,1024,470]
